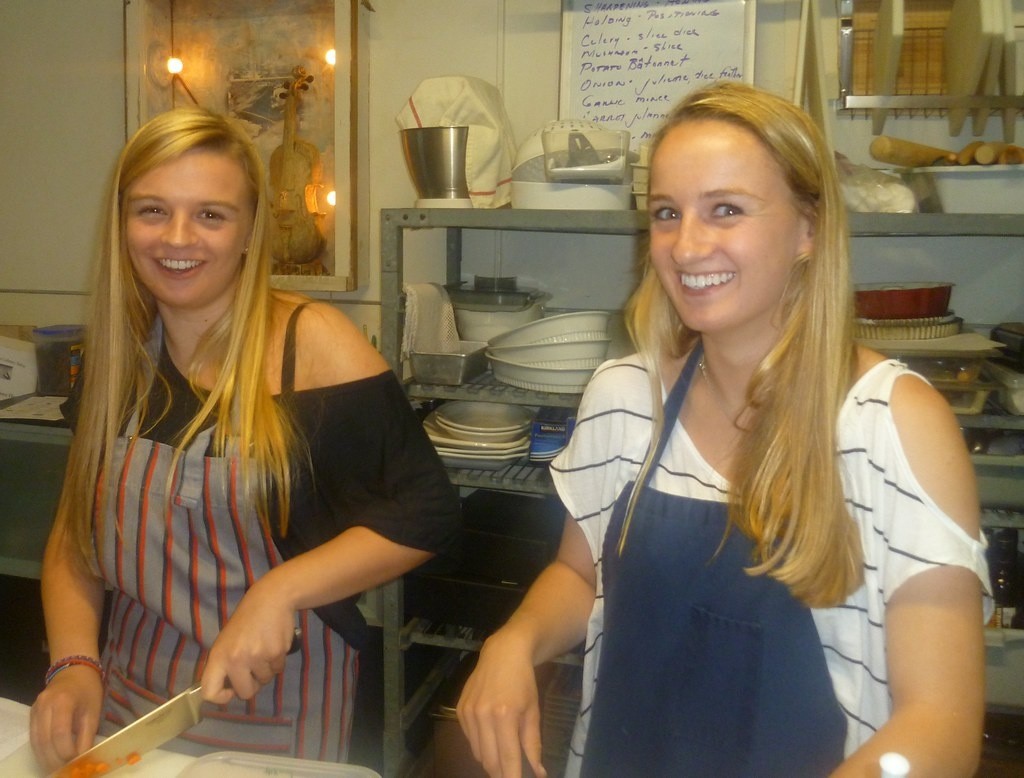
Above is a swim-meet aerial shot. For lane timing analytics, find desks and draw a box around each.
[1,697,292,778]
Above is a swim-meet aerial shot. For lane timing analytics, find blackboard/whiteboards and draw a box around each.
[560,1,758,156]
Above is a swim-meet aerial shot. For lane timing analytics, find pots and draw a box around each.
[449,274,554,341]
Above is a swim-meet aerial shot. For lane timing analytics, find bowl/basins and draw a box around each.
[510,180,633,210]
[853,281,956,319]
[484,311,613,394]
[400,126,471,200]
[893,164,1024,216]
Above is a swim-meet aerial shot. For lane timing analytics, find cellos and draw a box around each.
[265,66,327,271]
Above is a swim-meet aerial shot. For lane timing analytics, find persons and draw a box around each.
[456,82,997,778]
[29,107,461,774]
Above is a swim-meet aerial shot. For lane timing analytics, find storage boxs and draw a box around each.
[174,751,382,778]
[409,341,489,385]
[970,446,1024,507]
[428,653,581,777]
[33,325,87,396]
[984,627,1023,707]
[541,129,630,183]
[529,406,577,466]
[509,182,636,211]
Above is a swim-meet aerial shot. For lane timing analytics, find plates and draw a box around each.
[529,419,567,463]
[422,402,534,472]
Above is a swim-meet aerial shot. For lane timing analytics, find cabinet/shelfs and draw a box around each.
[380,1,1024,778]
[0,391,115,591]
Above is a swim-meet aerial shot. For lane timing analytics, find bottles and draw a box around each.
[986,531,1024,628]
[950,357,979,381]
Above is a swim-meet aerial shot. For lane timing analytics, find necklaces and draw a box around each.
[700,351,746,430]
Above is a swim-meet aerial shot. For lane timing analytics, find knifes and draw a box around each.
[44,627,303,778]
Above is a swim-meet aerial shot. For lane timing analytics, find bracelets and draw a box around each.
[46,656,106,688]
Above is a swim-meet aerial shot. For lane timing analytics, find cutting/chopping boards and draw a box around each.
[871,0,1017,145]
[2,697,196,778]
